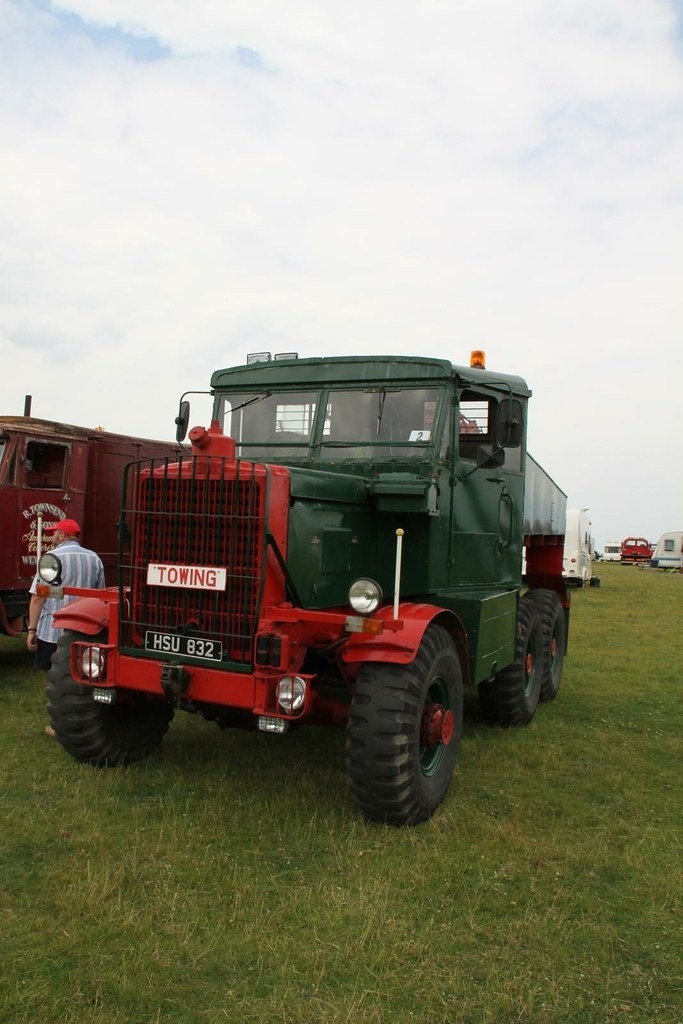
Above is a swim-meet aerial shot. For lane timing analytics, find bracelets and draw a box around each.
[28,627,37,633]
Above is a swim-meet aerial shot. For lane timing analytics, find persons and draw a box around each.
[26,519,106,739]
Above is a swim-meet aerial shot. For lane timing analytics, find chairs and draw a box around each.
[263,431,307,458]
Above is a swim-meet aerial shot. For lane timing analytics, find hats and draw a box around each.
[44,519,82,537]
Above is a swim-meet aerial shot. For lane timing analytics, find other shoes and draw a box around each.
[45,726,54,736]
